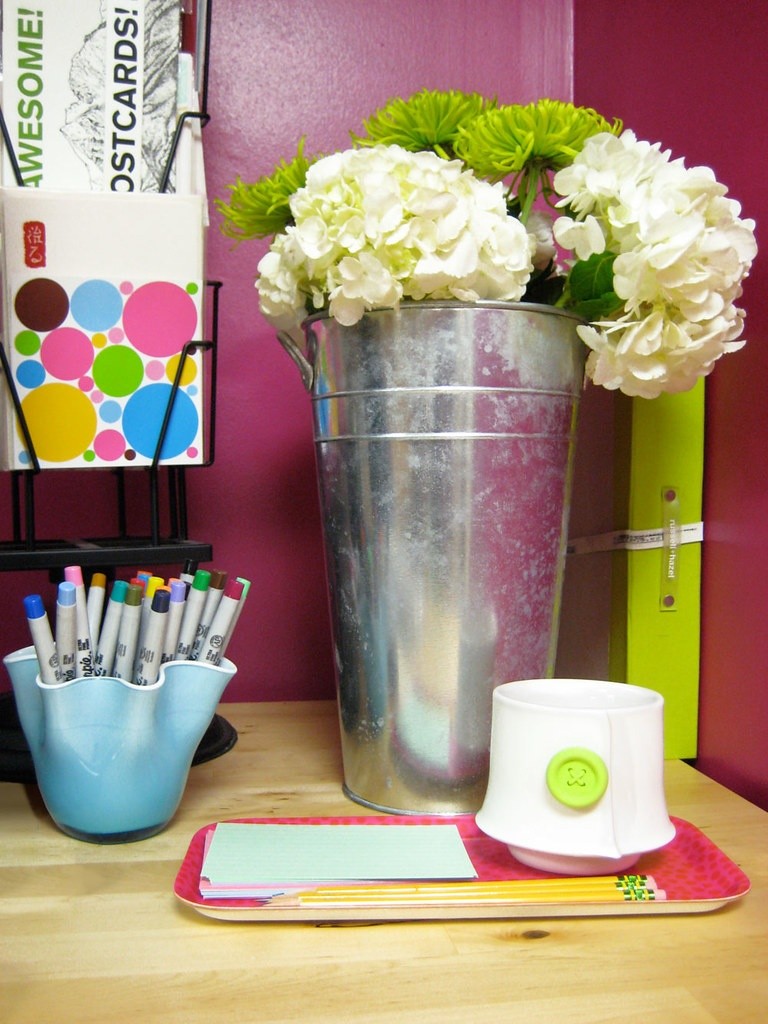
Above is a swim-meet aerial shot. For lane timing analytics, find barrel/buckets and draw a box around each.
[275,298,593,815]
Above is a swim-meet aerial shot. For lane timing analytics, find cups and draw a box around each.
[474,679,677,876]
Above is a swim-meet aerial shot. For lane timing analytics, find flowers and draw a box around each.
[213,87,759,400]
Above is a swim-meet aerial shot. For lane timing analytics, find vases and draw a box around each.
[297,301,586,817]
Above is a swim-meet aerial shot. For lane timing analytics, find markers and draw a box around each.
[22,557,251,686]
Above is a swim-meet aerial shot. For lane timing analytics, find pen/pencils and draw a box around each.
[255,875,666,908]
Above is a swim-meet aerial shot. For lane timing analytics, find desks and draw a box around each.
[0,701,768,1024]
